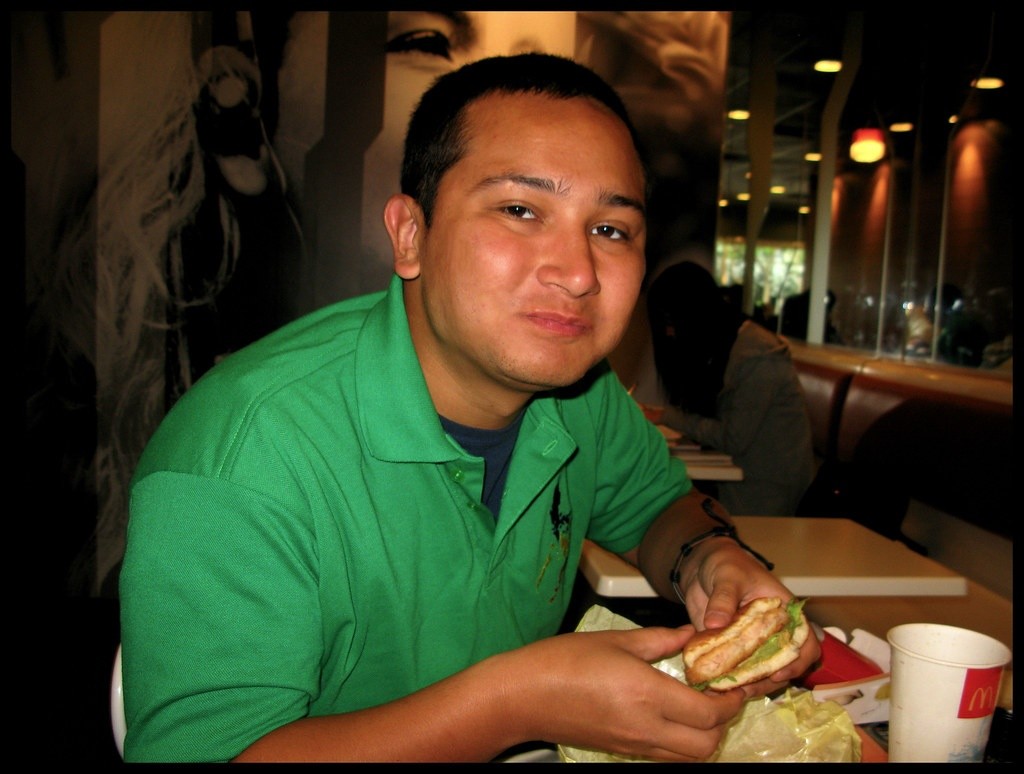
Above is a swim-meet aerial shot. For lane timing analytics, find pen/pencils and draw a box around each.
[627,381,636,394]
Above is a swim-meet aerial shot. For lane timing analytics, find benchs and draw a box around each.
[790,361,851,452]
[827,387,1012,571]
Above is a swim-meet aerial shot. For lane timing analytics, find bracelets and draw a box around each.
[670,498,774,604]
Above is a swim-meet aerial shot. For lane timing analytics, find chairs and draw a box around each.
[111,645,127,756]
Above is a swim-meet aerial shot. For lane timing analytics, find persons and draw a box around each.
[111,51,823,763]
[798,286,847,346]
[632,262,815,517]
[97,9,575,597]
[929,282,1013,369]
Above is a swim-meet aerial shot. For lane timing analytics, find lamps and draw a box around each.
[850,129,886,163]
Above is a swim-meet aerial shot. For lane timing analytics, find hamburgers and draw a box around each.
[682,597,809,693]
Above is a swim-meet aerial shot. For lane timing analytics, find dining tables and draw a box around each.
[670,440,744,481]
[581,519,967,599]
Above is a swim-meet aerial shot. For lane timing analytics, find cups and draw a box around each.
[887,623,1012,762]
